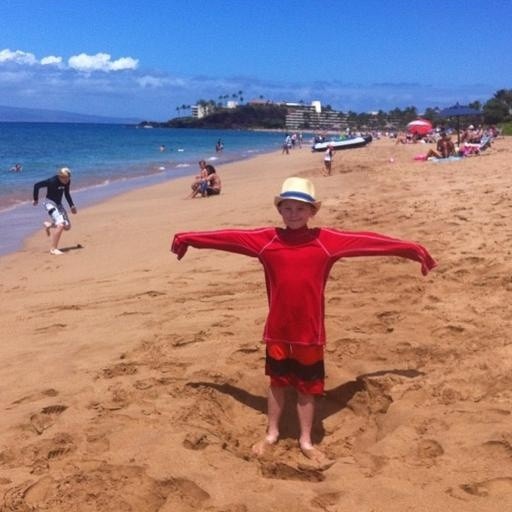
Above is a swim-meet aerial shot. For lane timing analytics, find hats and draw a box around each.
[272,175,321,209]
[59,166,71,178]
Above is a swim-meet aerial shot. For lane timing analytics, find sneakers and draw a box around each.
[48,248,62,256]
[43,221,51,236]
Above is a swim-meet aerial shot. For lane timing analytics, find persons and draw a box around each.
[198,160,208,198]
[220,141,224,150]
[187,165,222,199]
[281,122,498,159]
[216,141,222,153]
[324,144,333,175]
[170,176,438,460]
[160,145,165,152]
[33,167,78,255]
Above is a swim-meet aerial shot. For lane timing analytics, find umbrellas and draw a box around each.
[433,101,487,148]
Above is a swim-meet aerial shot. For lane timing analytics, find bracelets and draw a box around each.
[71,205,75,210]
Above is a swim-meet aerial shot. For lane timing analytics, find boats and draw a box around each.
[312,134,372,151]
